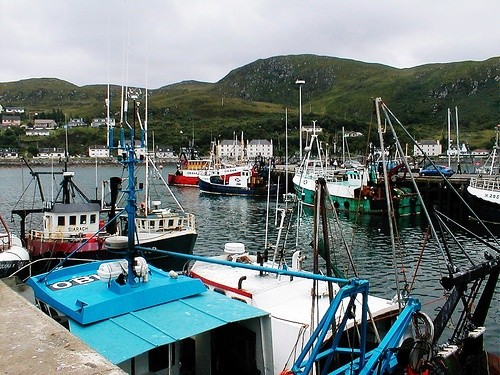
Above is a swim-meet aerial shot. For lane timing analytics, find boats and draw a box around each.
[0,38,500,374]
